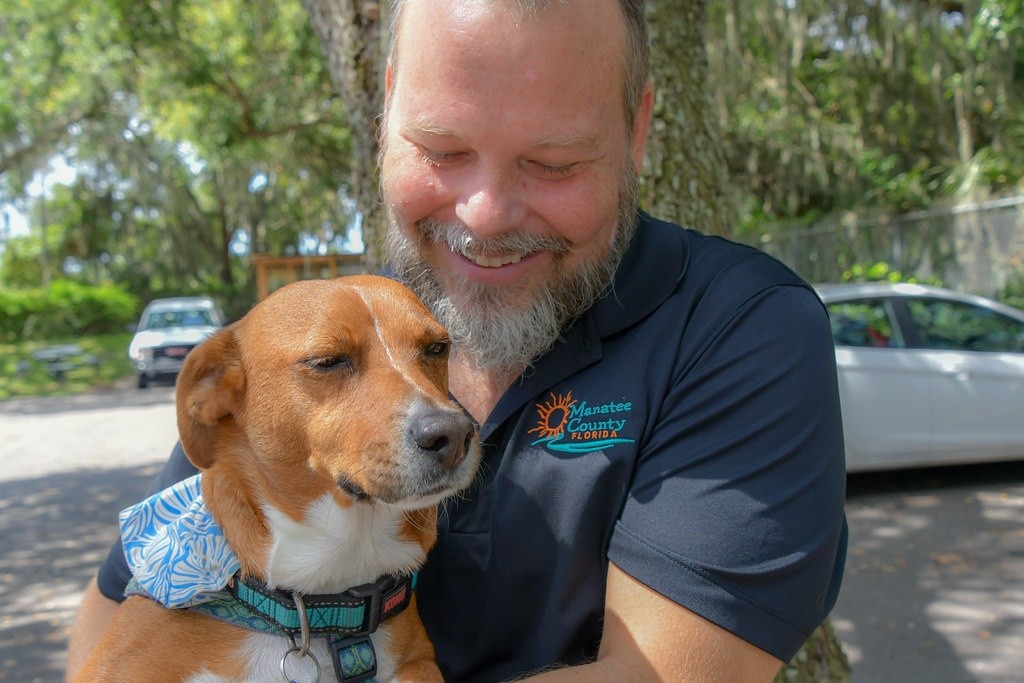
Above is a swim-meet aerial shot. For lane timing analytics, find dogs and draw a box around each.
[69,275,482,683]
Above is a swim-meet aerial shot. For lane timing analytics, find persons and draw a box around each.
[72,0,849,683]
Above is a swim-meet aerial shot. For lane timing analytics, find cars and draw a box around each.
[127,298,224,387]
[808,282,1024,471]
